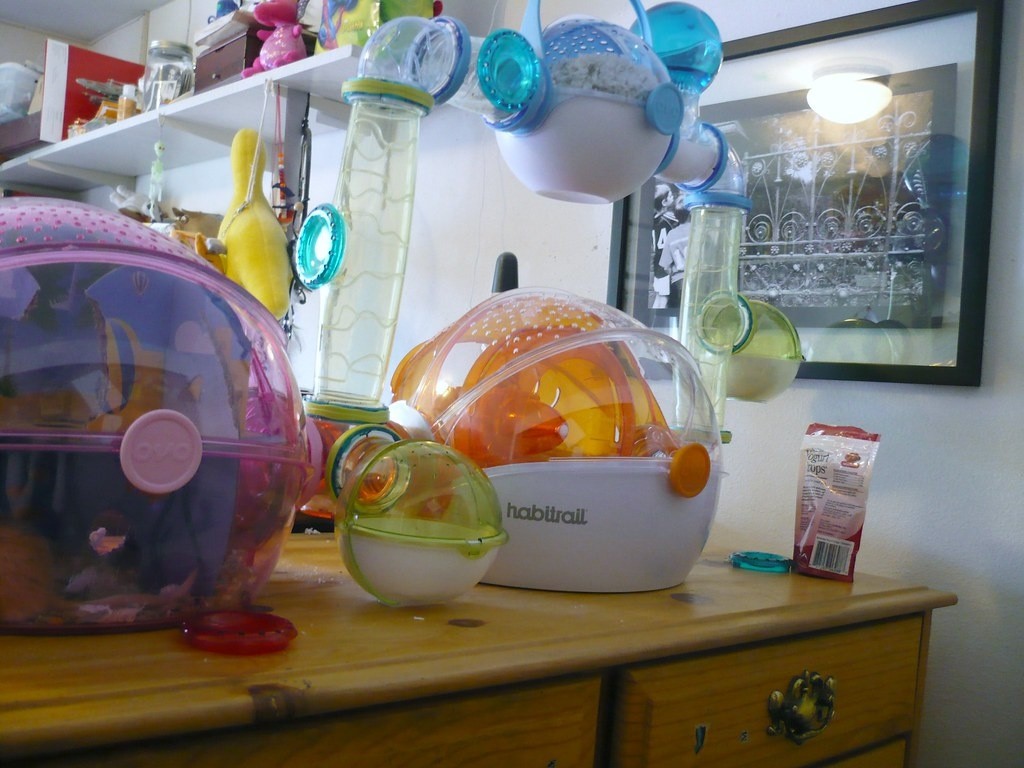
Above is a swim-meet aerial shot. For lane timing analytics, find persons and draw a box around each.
[143,63,180,110]
[648,183,693,309]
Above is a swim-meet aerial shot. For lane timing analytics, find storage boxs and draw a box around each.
[195,23,317,94]
[0,62,39,126]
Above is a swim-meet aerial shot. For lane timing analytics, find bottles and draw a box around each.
[143,40,194,113]
[117,83,138,122]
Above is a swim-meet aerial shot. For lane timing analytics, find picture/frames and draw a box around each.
[602,1,1005,390]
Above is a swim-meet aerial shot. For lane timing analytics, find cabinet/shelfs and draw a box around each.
[0,531,959,768]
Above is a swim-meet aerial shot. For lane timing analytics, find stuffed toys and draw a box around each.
[109,185,178,221]
[241,0,309,80]
[218,129,293,320]
